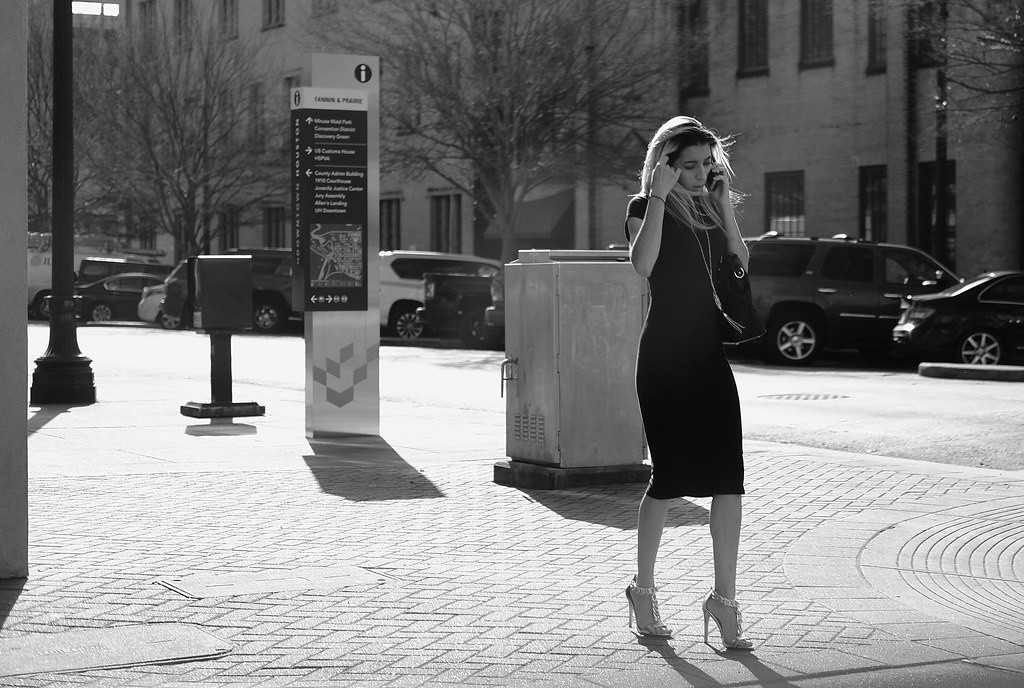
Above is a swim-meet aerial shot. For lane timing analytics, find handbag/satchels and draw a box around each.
[715,251,769,348]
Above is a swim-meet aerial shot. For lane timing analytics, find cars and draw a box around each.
[72,273,164,322]
[136,284,180,330]
[893,270,1024,367]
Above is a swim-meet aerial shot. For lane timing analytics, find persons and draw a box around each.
[624,111,757,653]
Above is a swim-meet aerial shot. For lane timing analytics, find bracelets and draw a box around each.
[647,193,666,205]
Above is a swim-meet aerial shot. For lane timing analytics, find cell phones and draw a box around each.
[705,169,720,192]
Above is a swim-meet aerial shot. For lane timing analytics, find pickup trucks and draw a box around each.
[413,266,507,351]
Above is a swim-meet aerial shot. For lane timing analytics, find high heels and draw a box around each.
[701,586,758,651]
[623,572,672,637]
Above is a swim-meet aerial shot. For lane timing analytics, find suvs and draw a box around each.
[27,230,129,320]
[79,256,175,286]
[742,229,966,365]
[379,249,507,342]
[162,257,209,333]
[225,247,300,333]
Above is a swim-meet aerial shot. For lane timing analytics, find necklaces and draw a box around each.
[670,189,746,335]
